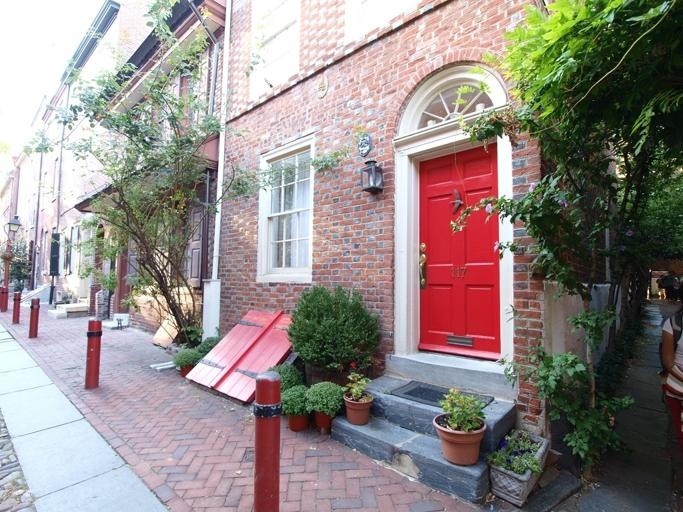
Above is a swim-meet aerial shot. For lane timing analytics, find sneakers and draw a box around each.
[670,489,683,512]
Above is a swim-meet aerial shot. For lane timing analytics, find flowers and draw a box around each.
[487,427,543,475]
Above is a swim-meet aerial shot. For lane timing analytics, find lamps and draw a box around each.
[361,157,384,193]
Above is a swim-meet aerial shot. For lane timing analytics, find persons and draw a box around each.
[657,309,683,454]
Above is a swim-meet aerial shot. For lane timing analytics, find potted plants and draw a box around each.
[433,386,487,467]
[265,286,382,434]
[174,349,200,378]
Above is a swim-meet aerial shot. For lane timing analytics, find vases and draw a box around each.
[488,430,552,508]
